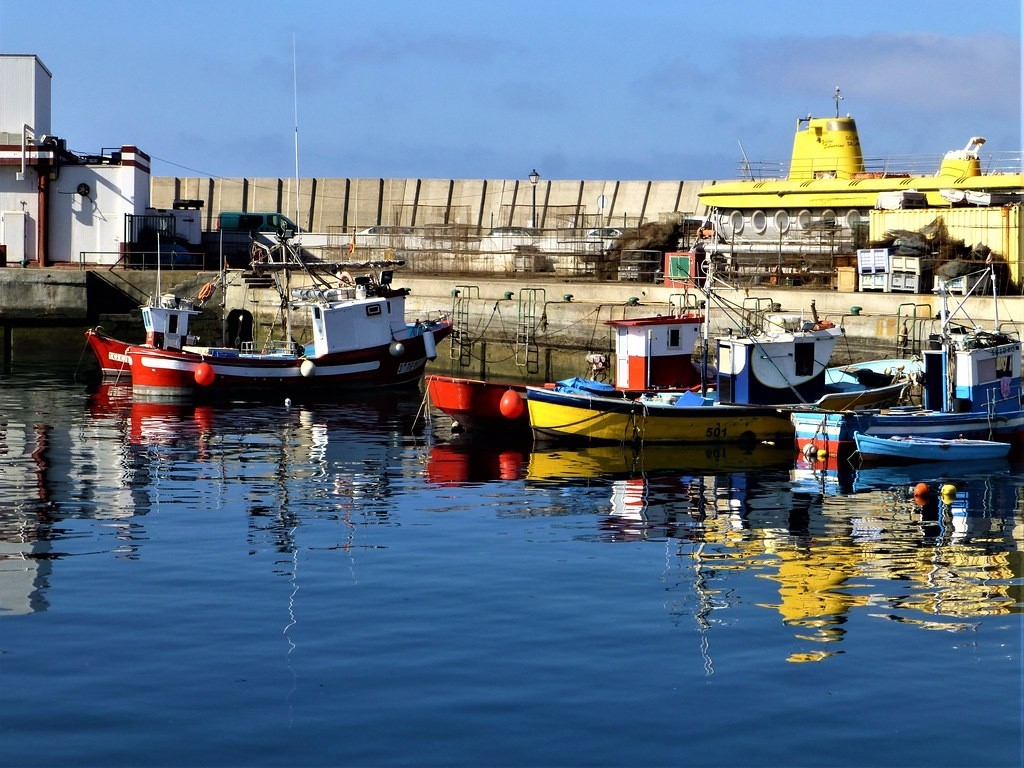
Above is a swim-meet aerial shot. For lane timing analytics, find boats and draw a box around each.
[523,429,791,492]
[792,268,1020,459]
[696,84,1024,274]
[522,249,923,442]
[423,312,717,437]
[854,430,1012,460]
[852,461,1011,494]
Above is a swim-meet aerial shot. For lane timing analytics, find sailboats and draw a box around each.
[122,32,453,397]
[788,452,870,495]
[83,229,204,377]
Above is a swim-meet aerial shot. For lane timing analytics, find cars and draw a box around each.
[489,227,542,237]
[584,226,632,251]
[356,227,413,234]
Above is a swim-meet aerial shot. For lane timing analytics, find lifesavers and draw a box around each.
[338,271,354,289]
[198,282,211,299]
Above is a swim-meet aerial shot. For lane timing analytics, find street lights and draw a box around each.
[528,169,540,228]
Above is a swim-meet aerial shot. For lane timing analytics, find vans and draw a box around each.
[216,212,309,234]
